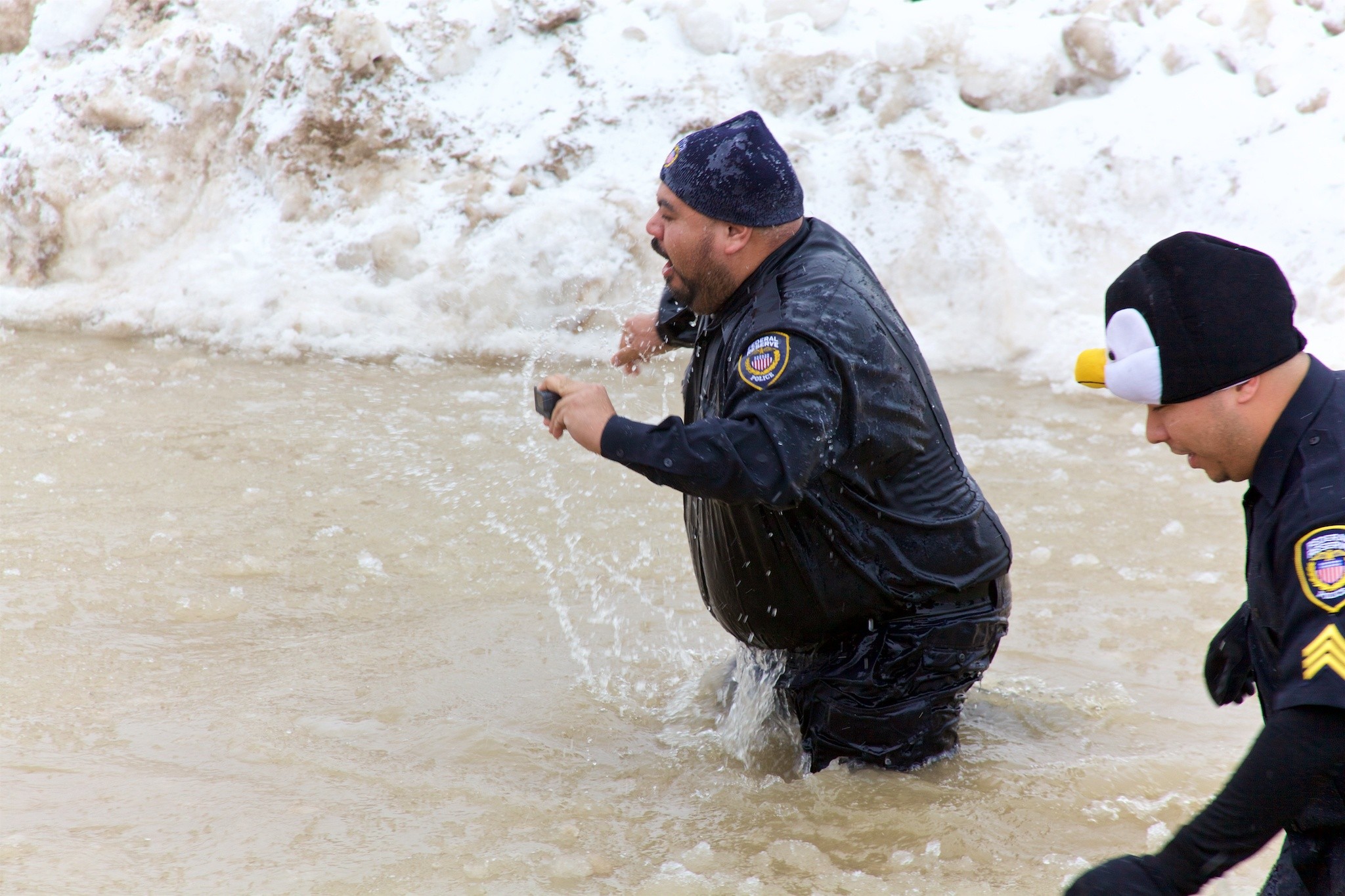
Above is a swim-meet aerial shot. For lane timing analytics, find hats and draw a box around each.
[1074,231,1307,404]
[658,111,804,226]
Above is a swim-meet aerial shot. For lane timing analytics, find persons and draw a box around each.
[538,112,1012,777]
[1063,231,1345,896]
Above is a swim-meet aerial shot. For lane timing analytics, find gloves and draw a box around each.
[1065,853,1186,895]
[1204,603,1259,707]
